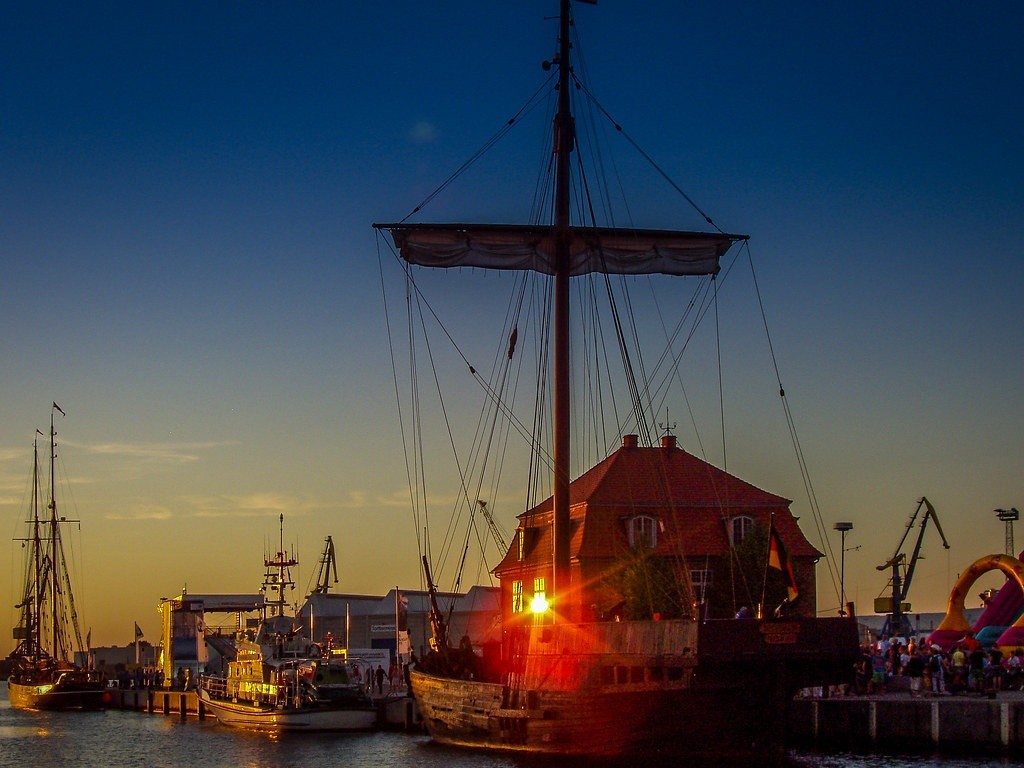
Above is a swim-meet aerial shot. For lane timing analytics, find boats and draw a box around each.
[378,529,462,735]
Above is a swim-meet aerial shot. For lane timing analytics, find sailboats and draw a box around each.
[370,1,857,766]
[8,402,120,713]
[194,513,381,735]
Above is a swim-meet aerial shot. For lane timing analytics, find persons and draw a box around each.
[237,629,293,659]
[167,667,226,699]
[275,678,307,708]
[734,602,755,619]
[80,665,165,690]
[352,663,404,694]
[852,634,1024,698]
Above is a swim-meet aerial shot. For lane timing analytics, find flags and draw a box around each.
[87,631,91,650]
[135,624,144,640]
[397,594,413,653]
